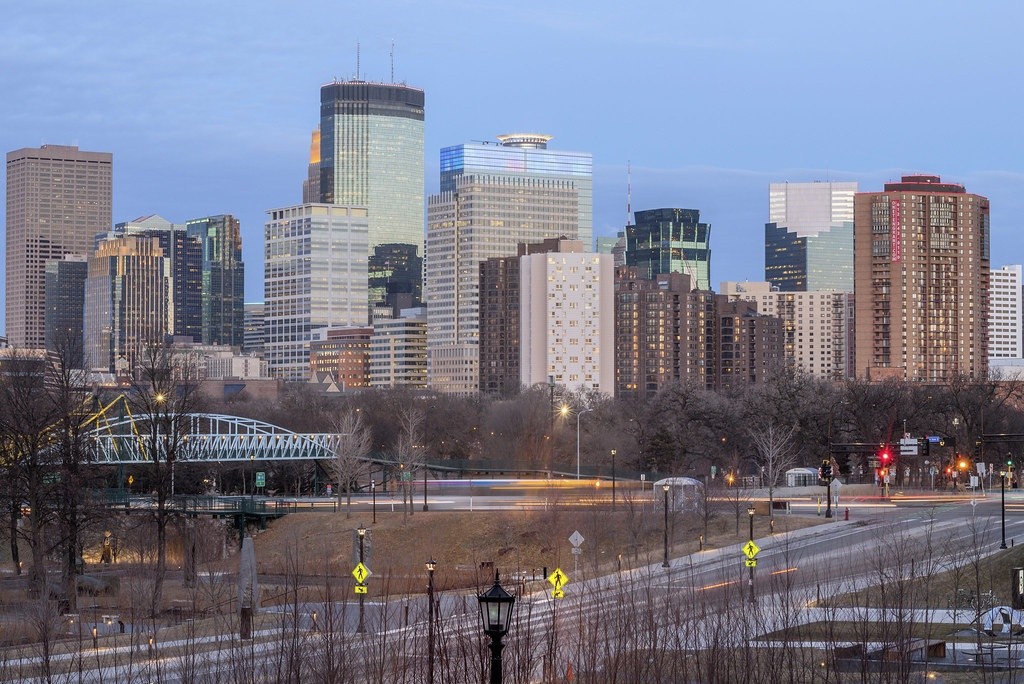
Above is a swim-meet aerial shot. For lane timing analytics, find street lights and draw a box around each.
[662,481,670,567]
[576,409,593,480]
[424,405,436,512]
[477,567,516,684]
[610,447,617,512]
[548,383,555,454]
[357,523,366,633]
[424,555,438,684]
[746,503,756,602]
[998,464,1008,549]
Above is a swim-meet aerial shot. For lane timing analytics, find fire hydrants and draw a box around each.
[844,507,849,520]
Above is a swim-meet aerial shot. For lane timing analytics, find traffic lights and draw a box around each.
[945,467,953,474]
[960,461,967,469]
[1007,454,1012,465]
[880,450,893,463]
[844,457,853,474]
[821,463,832,479]
[880,470,887,477]
